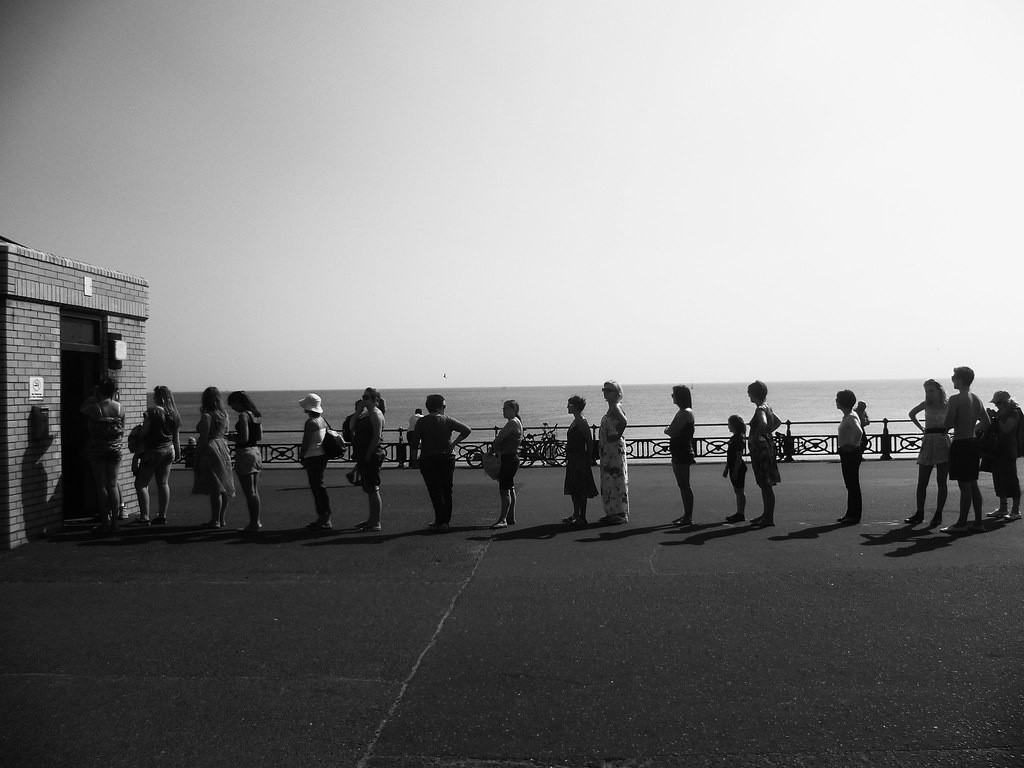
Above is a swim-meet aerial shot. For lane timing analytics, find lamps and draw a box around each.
[112,339,127,360]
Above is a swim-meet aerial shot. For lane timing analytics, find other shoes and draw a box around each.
[560,503,1022,531]
[491,519,508,529]
[199,521,226,528]
[235,522,263,532]
[306,517,333,530]
[506,517,517,525]
[91,525,118,533]
[419,522,450,534]
[119,506,130,520]
[352,520,382,532]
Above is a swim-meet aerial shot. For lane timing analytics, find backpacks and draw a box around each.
[340,413,356,443]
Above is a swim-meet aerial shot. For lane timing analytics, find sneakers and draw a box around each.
[126,518,153,527]
[149,517,168,526]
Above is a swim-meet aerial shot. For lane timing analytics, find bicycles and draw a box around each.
[516,422,571,467]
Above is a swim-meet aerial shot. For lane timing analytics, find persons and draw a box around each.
[598,379,629,524]
[226,390,263,531]
[561,395,597,526]
[132,385,181,527]
[904,367,1024,533]
[299,392,333,530]
[407,408,426,466]
[836,388,870,526]
[746,380,781,528]
[80,377,125,534]
[192,386,237,529]
[413,394,471,527]
[489,399,524,527]
[663,385,696,529]
[342,388,385,531]
[723,415,747,521]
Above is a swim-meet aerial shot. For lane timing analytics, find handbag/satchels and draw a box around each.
[321,417,347,460]
[755,406,781,435]
[237,410,261,441]
[127,423,151,457]
[974,418,1002,458]
[481,444,503,481]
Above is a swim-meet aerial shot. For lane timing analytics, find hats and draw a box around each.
[990,391,1010,404]
[297,393,323,413]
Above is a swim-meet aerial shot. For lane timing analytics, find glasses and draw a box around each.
[952,375,958,381]
[361,395,371,401]
[603,388,615,393]
[924,379,936,386]
